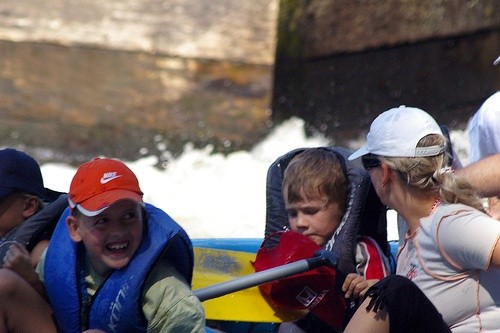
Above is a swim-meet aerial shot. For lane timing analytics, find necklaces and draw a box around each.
[407,193,441,235]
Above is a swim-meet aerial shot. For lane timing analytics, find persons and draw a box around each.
[0,155,206,333]
[0,147,69,269]
[341,104,500,332]
[277,148,391,333]
[448,91,500,220]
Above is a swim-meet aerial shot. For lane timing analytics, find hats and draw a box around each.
[68,157,145,217]
[0,148,68,202]
[347,105,448,161]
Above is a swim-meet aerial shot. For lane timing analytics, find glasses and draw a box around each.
[362,155,381,170]
